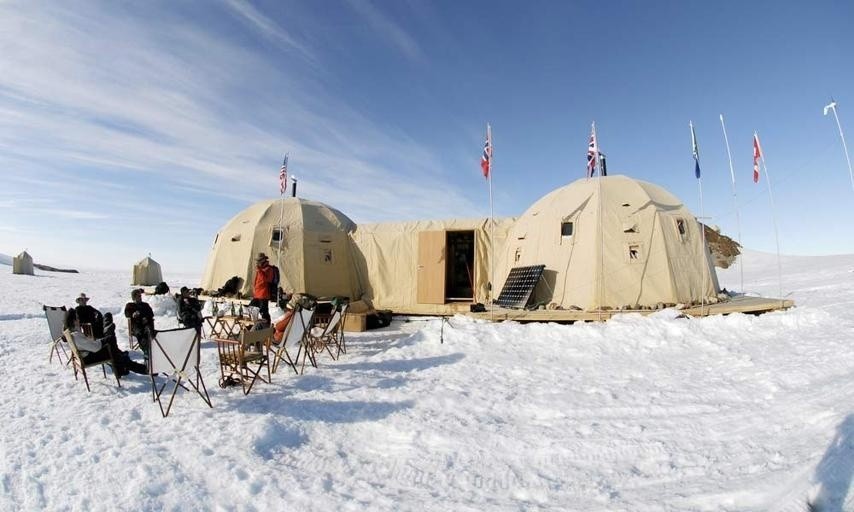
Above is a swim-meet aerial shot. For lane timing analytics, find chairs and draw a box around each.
[60,321,121,392]
[253,296,348,375]
[215,325,274,394]
[42,304,73,364]
[128,314,146,352]
[145,319,212,417]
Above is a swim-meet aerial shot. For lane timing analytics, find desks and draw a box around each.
[204,313,265,343]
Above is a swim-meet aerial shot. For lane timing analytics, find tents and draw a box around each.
[489,174,722,310]
[198,197,373,309]
[11,250,33,274]
[130,256,162,286]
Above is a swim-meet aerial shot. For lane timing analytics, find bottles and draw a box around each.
[238,301,243,318]
[230,301,235,317]
[212,301,224,319]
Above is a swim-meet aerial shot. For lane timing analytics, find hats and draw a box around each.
[75,294,89,303]
[180,287,190,294]
[254,253,268,261]
[285,293,309,310]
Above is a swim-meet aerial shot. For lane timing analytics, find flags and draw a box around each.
[586,124,597,179]
[752,135,760,183]
[479,124,492,178]
[690,124,700,181]
[277,152,288,195]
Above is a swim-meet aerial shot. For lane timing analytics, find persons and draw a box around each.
[176,286,203,342]
[73,293,117,348]
[64,308,158,377]
[245,253,273,326]
[234,292,305,360]
[123,289,155,360]
